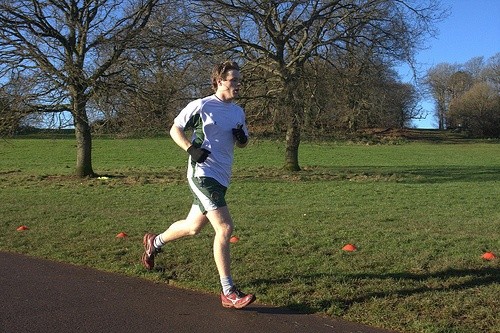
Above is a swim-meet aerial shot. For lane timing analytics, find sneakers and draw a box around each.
[141,233,163,270]
[220,283,256,309]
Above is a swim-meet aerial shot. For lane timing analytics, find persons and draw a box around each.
[142,61,256,308]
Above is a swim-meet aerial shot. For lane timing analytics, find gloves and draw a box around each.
[232,124,248,144]
[187,145,211,163]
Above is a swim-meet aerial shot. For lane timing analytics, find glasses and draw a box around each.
[220,60,240,74]
